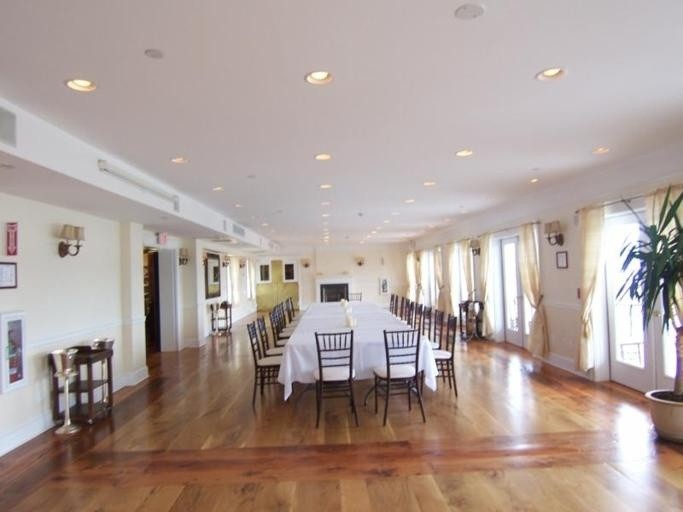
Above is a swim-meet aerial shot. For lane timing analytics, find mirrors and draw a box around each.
[203,252,220,300]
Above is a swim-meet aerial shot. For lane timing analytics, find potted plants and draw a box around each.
[615,183,682,444]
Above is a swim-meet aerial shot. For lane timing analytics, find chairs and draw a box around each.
[246,292,457,429]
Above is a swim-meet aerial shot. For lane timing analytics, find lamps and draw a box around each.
[58,223,84,259]
[469,238,480,256]
[542,219,564,246]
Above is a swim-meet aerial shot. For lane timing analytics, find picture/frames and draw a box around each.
[555,251,568,269]
[0,261,17,288]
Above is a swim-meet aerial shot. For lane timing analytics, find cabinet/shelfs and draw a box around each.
[209,303,231,334]
[47,345,113,428]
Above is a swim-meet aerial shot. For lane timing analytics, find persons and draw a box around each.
[8,330,17,355]
